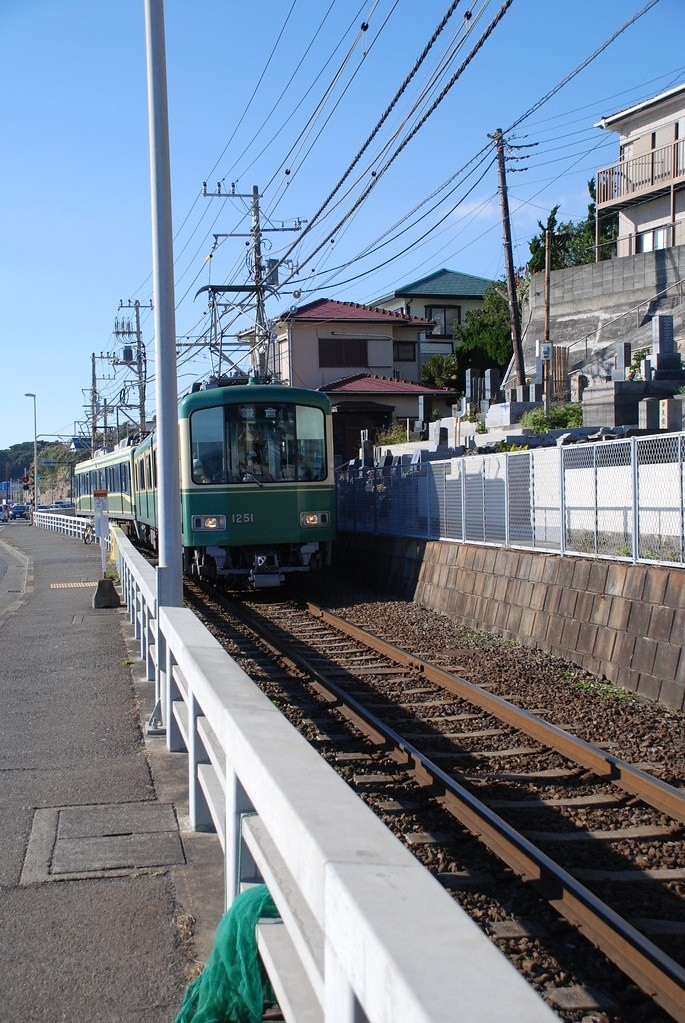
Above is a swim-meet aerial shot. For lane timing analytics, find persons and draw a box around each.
[28,498,36,525]
[192,459,210,484]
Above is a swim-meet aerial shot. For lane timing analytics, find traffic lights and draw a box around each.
[22,483,29,490]
[23,477,29,484]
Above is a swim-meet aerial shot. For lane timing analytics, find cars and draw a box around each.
[0,500,74,523]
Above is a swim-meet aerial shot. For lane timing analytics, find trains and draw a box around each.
[71,376,339,594]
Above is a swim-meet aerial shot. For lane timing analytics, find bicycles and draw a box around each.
[82,515,95,546]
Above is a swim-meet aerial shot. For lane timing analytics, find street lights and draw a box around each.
[24,392,39,512]
[495,286,527,386]
